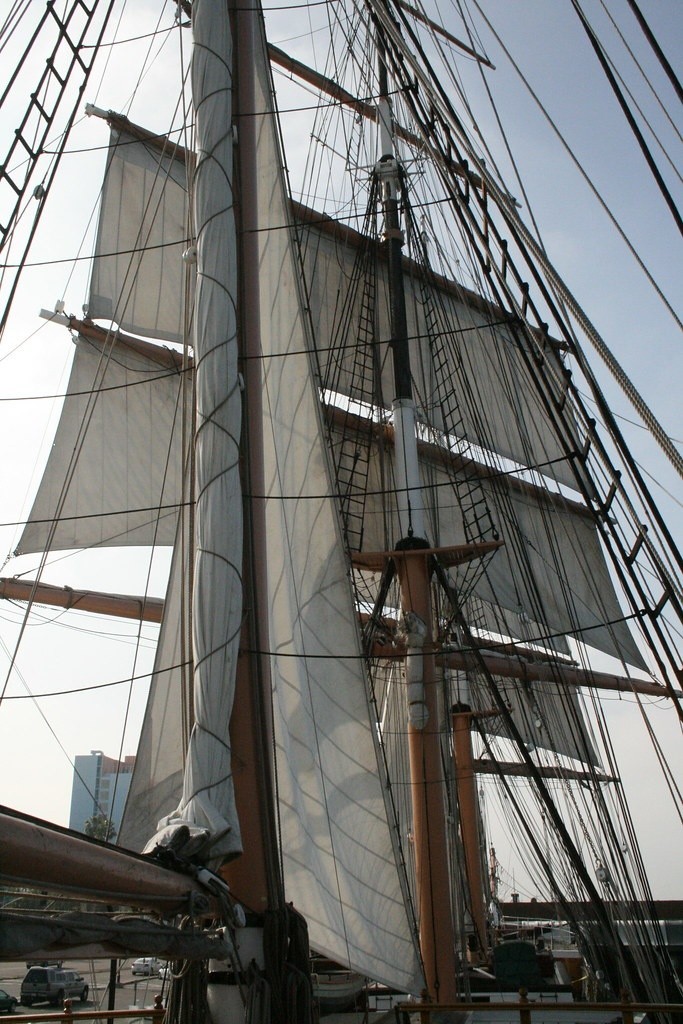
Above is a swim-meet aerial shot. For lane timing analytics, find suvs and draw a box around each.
[20,966,89,1006]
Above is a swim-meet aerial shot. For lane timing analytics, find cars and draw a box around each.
[131,959,162,975]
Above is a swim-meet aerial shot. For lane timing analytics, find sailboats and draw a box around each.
[0,0,682,1024]
